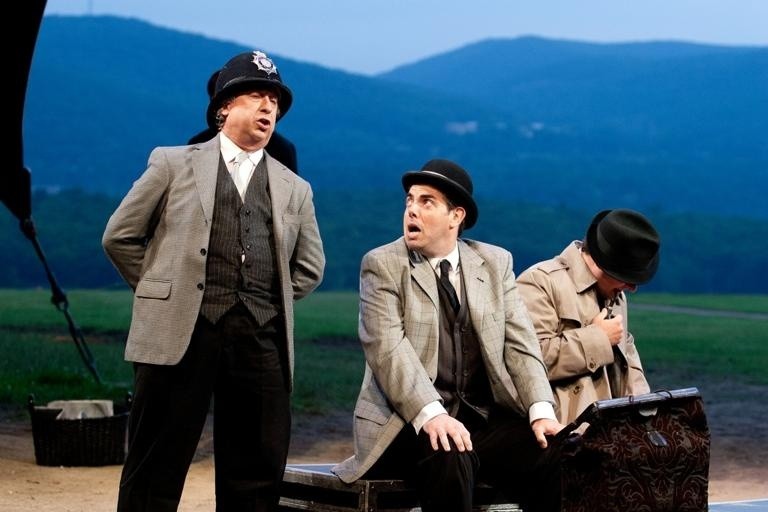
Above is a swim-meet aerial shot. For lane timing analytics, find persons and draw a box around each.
[102,50,326,512]
[330,158,564,512]
[515,208,660,425]
[187,72,297,174]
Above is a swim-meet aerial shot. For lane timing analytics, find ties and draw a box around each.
[230,151,249,204]
[440,259,460,315]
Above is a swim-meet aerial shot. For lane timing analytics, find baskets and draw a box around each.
[28,391,133,467]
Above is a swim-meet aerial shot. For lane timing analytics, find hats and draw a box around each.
[586,208,660,285]
[207,51,292,128]
[402,159,477,229]
